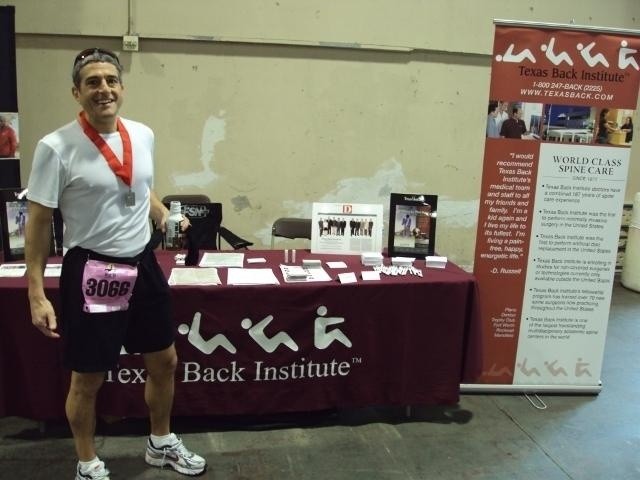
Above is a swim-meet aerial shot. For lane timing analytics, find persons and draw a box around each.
[0,115,16,158]
[598,108,618,144]
[486,101,527,140]
[620,116,633,143]
[319,216,373,237]
[402,215,412,237]
[23,47,206,480]
[16,210,26,239]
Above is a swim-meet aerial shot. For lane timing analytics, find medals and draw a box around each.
[124,187,136,207]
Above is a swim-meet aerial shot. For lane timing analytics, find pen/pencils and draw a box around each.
[373,264,423,278]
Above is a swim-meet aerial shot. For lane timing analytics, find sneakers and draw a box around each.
[74,457,109,479]
[144,433,207,476]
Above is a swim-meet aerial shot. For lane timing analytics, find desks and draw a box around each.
[547,126,628,147]
[0,246,483,437]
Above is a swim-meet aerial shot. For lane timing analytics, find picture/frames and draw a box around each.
[308,200,384,258]
[0,187,57,262]
[387,190,439,259]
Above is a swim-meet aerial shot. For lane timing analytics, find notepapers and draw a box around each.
[326,260,348,269]
[247,257,267,264]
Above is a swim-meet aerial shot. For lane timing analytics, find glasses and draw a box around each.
[70,48,121,64]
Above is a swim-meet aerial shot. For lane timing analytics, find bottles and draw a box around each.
[292,250,296,263]
[162,199,185,251]
[285,250,289,264]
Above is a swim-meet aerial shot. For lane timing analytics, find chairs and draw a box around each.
[268,217,312,250]
[145,193,253,253]
